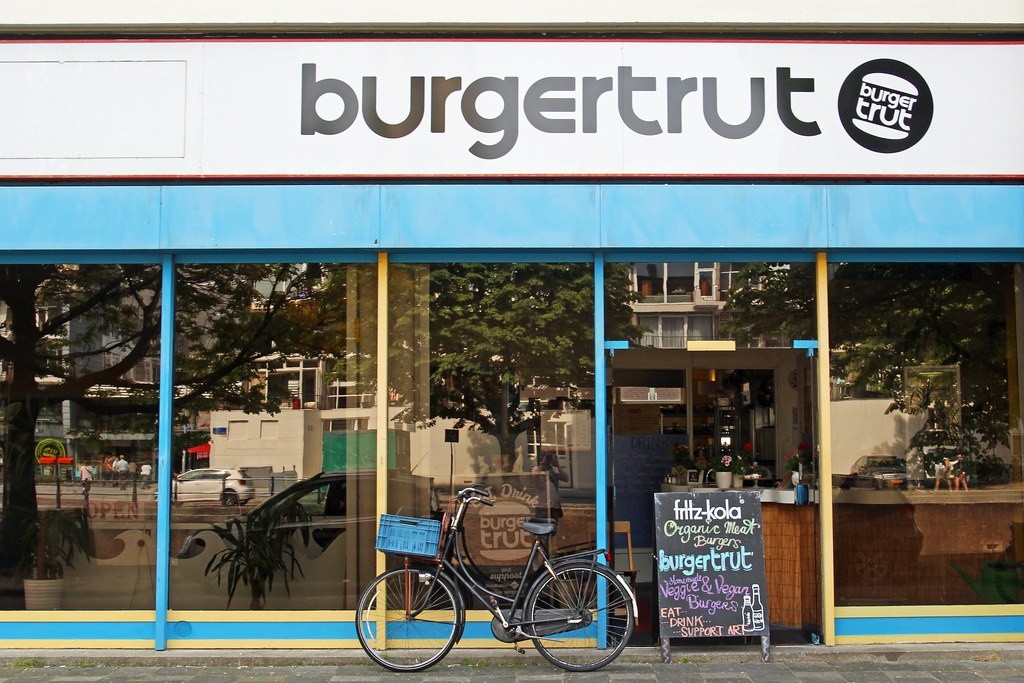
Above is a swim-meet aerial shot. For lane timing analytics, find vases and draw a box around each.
[733,473,743,488]
[715,472,732,490]
[791,470,799,486]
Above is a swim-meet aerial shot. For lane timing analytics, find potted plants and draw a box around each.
[0,507,91,610]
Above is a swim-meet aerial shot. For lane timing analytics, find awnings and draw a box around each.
[187,443,211,452]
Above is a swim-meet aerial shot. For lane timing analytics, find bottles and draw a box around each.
[667,474,671,484]
[671,474,678,484]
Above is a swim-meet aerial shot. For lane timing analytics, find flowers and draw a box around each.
[710,445,749,473]
[783,442,810,472]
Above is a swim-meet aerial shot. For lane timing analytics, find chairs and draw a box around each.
[586,520,640,626]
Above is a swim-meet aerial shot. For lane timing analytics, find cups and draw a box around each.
[733,474,743,489]
[716,472,732,488]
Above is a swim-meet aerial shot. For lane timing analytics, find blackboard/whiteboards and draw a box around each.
[654,490,770,638]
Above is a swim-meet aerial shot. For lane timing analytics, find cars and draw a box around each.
[156,468,257,508]
[906,444,991,492]
[153,468,561,548]
[850,455,908,491]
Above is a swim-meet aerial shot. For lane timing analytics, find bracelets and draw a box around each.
[540,462,543,465]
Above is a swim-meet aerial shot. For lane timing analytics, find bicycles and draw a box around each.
[355,486,639,673]
[397,492,596,610]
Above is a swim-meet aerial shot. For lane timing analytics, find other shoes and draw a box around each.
[83,490,85,494]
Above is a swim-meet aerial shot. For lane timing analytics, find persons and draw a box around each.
[80,451,153,500]
[531,445,570,561]
[929,441,969,495]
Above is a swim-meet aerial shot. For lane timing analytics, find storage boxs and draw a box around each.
[321,429,411,474]
